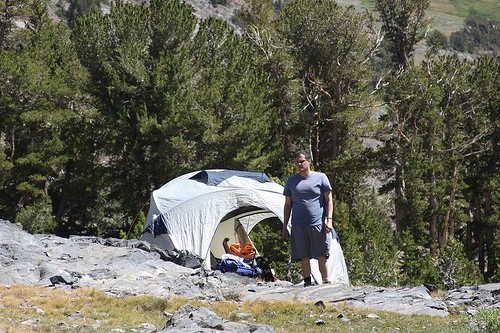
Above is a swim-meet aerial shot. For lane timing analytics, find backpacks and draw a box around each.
[215,242,277,283]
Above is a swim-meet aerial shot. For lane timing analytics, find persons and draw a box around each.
[280,149,334,286]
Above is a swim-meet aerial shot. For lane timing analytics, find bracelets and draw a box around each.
[327,218,333,220]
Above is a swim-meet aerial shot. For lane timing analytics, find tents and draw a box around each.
[125,168,349,286]
